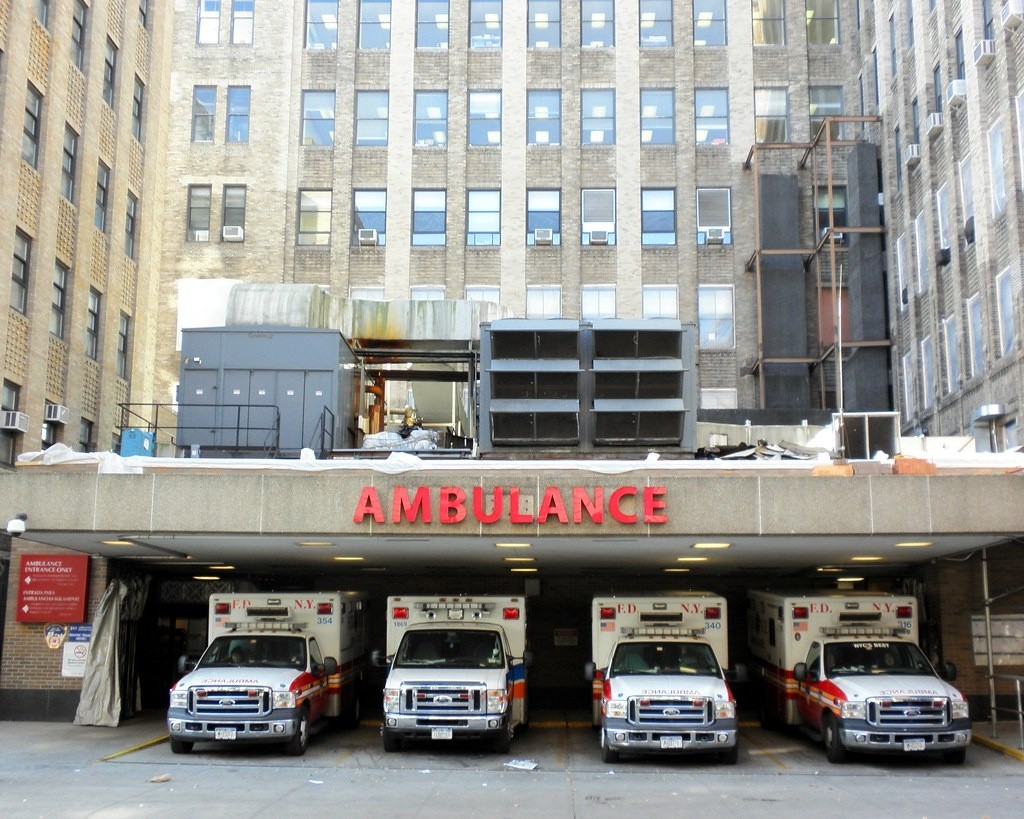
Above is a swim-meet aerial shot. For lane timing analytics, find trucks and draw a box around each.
[743,588,973,766]
[584,589,749,766]
[384,595,532,754]
[165,590,370,758]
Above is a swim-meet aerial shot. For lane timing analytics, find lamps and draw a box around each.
[6,514,29,538]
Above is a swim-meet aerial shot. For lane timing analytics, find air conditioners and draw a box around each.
[1001,0,1024,33]
[904,144,922,166]
[820,228,843,243]
[0,411,30,434]
[705,228,726,245]
[44,404,70,426]
[973,40,996,67]
[534,229,553,246]
[948,79,967,109]
[589,230,608,246]
[358,228,378,247]
[923,111,942,137]
[222,226,244,243]
